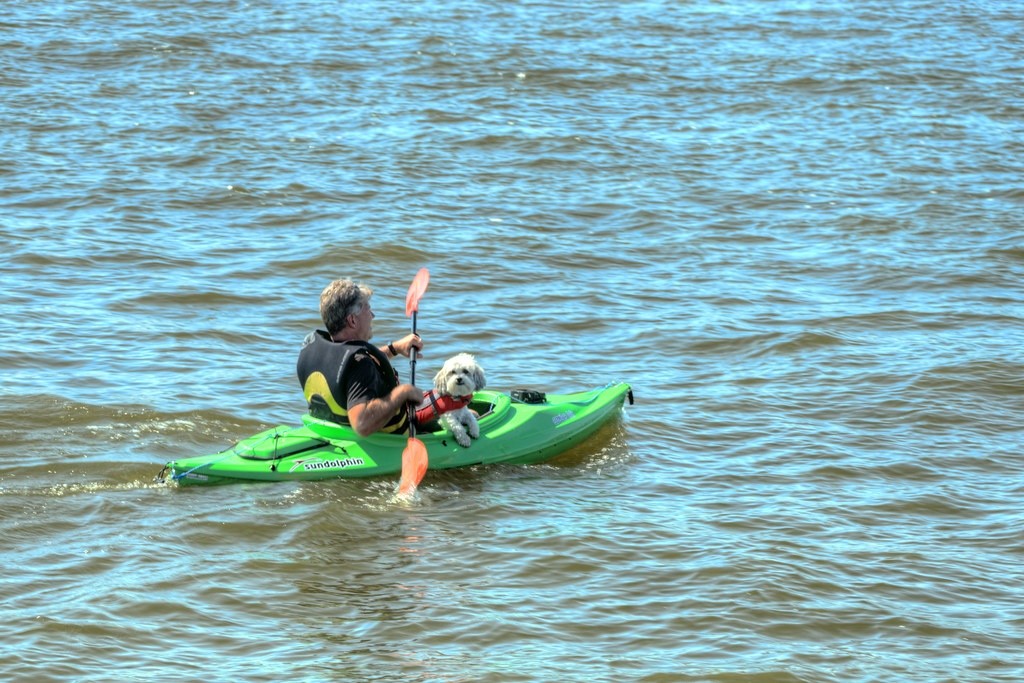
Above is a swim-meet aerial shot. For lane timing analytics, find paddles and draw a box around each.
[396,266,432,494]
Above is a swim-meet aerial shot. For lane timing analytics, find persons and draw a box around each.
[296,279,424,438]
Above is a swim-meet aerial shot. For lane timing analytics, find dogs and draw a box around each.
[423,352,486,448]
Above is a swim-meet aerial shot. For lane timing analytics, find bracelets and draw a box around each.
[388,342,398,356]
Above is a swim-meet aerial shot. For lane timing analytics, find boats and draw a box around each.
[154,381,634,485]
